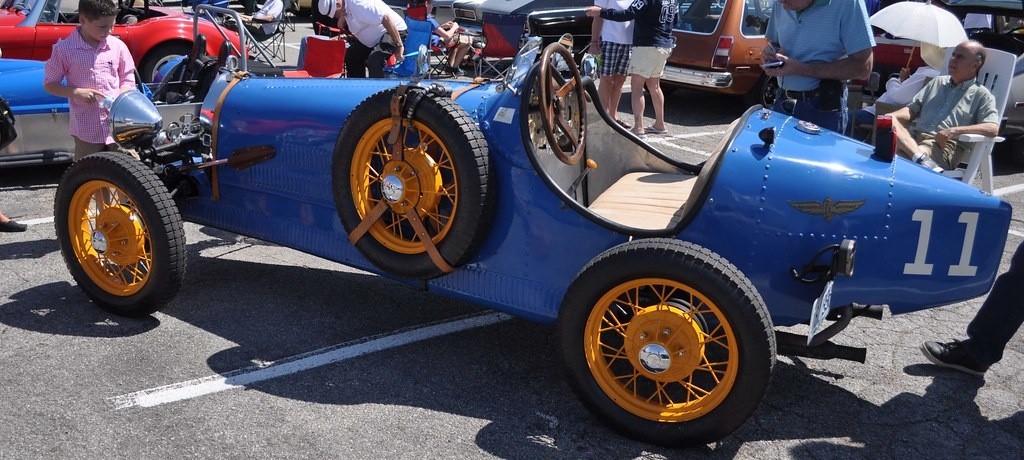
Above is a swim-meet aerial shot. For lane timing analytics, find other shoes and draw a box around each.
[445,65,467,76]
[916,152,944,174]
[616,118,632,128]
[0,221,27,231]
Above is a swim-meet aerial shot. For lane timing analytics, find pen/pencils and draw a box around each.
[767,42,776,53]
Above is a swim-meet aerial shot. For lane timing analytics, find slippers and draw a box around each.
[629,129,646,138]
[645,125,668,134]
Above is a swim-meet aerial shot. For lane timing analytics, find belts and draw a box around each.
[779,88,821,100]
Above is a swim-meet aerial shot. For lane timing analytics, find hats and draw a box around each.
[318,0,336,18]
[920,41,946,70]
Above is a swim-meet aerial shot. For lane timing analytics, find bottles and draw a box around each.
[94,94,114,111]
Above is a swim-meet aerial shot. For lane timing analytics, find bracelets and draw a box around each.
[396,42,402,46]
[592,41,597,42]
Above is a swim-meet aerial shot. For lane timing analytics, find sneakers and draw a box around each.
[919,339,986,375]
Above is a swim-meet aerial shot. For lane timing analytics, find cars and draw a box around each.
[50,33,1014,450]
[0,0,1024,178]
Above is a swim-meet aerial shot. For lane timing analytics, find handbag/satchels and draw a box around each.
[0,98,17,150]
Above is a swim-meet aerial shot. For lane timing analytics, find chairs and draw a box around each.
[851,45,1016,198]
[385,17,431,79]
[469,12,528,78]
[284,37,345,80]
[222,0,286,62]
[586,118,742,230]
[406,5,454,79]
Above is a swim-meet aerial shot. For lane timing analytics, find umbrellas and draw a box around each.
[869,0,970,66]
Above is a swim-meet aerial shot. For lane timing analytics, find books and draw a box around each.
[762,62,782,67]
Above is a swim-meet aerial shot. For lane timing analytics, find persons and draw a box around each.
[845,42,946,135]
[885,40,999,174]
[223,0,283,42]
[44,0,136,212]
[585,0,679,138]
[318,0,408,79]
[760,0,877,136]
[415,0,473,75]
[0,213,27,232]
[922,239,1024,376]
[588,0,634,129]
